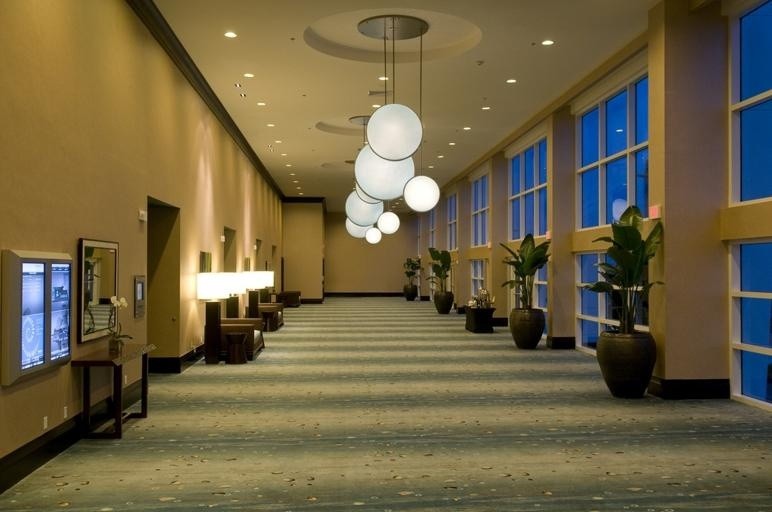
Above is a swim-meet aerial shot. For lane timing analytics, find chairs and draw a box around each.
[245,302,284,332]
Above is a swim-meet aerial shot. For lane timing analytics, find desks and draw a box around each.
[463,304,497,333]
[71,342,156,437]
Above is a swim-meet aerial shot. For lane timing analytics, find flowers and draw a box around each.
[108,296,127,334]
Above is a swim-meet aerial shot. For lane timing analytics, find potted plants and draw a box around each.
[583,204,664,399]
[425,247,454,314]
[402,257,422,300]
[499,233,552,349]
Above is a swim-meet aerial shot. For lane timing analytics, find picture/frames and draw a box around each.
[78,237,121,342]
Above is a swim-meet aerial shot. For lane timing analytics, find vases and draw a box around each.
[109,335,123,355]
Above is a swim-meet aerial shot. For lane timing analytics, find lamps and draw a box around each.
[196,270,275,365]
[345,15,440,243]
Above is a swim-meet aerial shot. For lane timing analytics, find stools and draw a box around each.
[279,291,301,307]
